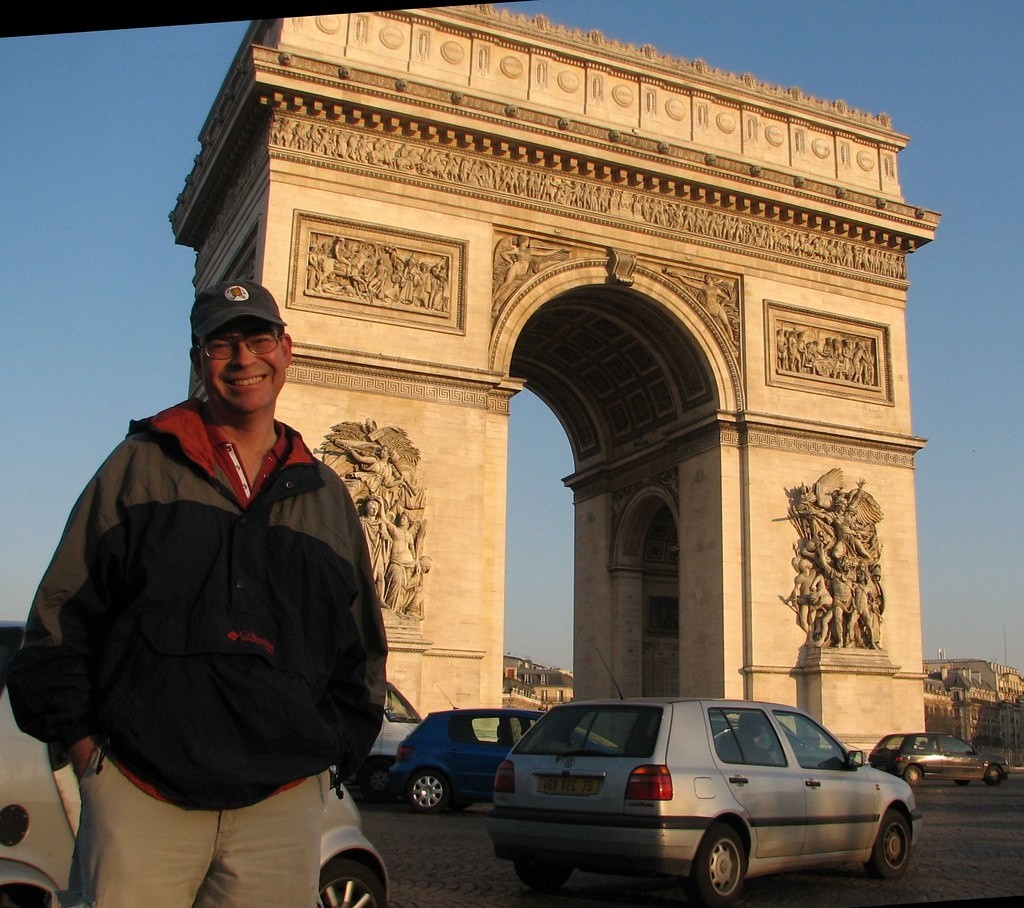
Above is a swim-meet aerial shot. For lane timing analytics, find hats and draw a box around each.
[190,280,288,337]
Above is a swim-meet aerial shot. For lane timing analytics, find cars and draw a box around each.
[487,698,924,908]
[868,732,1010,790]
[0,618,392,908]
[387,708,547,816]
[343,681,423,804]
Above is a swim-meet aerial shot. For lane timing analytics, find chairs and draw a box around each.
[718,734,772,764]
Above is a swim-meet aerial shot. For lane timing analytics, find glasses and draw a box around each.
[196,333,278,360]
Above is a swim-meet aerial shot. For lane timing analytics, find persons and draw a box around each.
[738,715,763,743]
[343,444,424,614]
[6,280,389,908]
[791,479,877,649]
